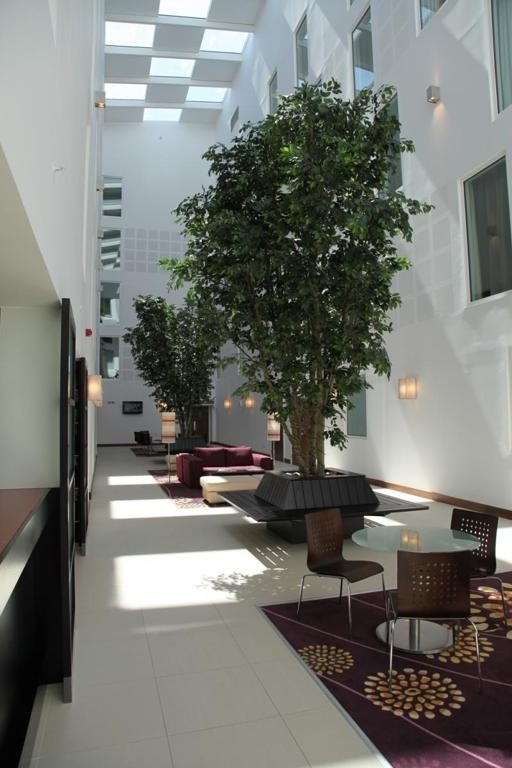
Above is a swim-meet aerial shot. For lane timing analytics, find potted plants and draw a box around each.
[160,74,434,545]
[121,288,222,450]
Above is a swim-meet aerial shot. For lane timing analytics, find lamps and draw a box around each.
[266,411,281,473]
[161,412,175,483]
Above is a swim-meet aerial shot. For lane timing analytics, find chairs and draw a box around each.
[134,431,153,455]
[296,506,505,689]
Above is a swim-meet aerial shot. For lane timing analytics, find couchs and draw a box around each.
[175,445,273,489]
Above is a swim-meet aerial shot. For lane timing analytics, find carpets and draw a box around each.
[146,469,205,507]
[131,448,167,457]
[256,569,511,768]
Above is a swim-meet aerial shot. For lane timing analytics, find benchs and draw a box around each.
[217,489,429,545]
[200,473,275,504]
